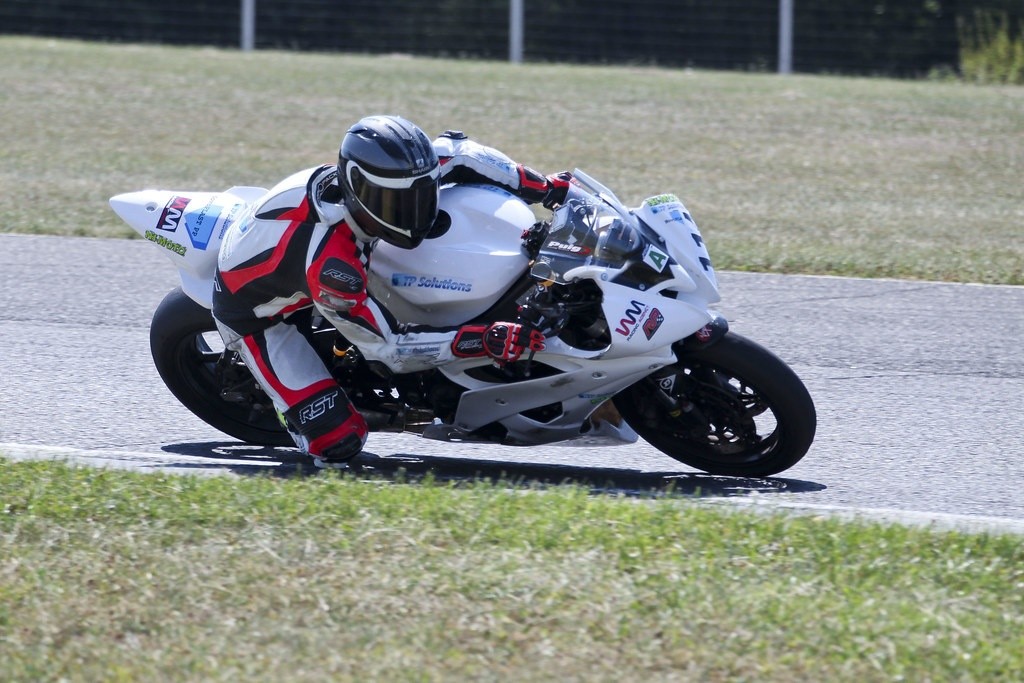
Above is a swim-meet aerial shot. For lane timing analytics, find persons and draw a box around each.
[211,110,579,471]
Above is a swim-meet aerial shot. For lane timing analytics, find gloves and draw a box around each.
[451,321,547,365]
[516,163,572,211]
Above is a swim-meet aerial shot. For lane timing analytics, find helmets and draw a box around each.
[339,115,441,251]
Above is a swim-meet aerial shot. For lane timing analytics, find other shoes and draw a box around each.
[273,404,308,456]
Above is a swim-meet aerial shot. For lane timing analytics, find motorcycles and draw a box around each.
[109,167,818,479]
